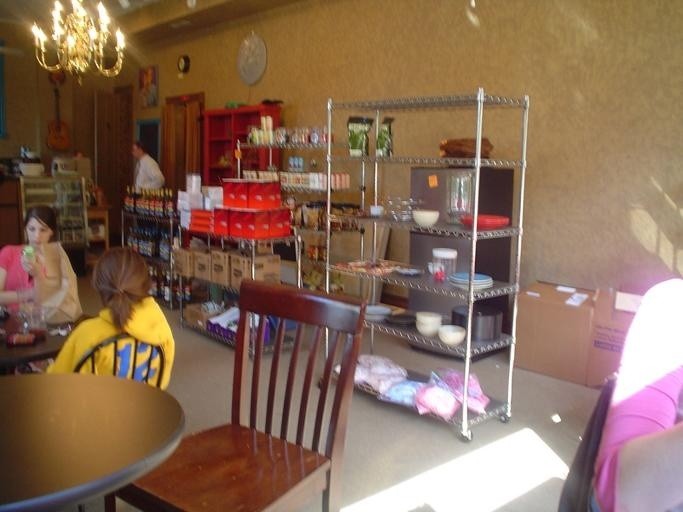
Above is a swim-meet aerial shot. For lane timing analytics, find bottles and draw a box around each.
[155,188,160,217]
[160,232,164,259]
[143,230,147,256]
[184,276,192,302]
[23,246,35,265]
[176,275,183,302]
[288,156,304,172]
[266,117,273,129]
[164,188,169,217]
[125,186,130,212]
[251,128,333,144]
[130,186,135,213]
[136,187,141,209]
[139,230,143,254]
[150,188,155,215]
[159,189,164,218]
[163,233,169,262]
[160,270,164,297]
[261,117,267,129]
[133,226,139,253]
[154,266,159,297]
[154,230,158,255]
[167,189,173,218]
[144,188,149,215]
[164,271,172,304]
[141,187,145,214]
[127,226,133,251]
[280,172,350,190]
[148,266,154,296]
[147,230,153,257]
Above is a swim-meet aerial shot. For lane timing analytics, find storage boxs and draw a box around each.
[514,280,598,386]
[587,286,646,389]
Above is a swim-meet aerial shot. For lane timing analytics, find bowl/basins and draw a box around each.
[414,311,444,338]
[412,210,441,230]
[364,303,392,324]
[437,325,468,346]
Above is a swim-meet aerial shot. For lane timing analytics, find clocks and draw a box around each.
[177,55,190,73]
[238,35,267,83]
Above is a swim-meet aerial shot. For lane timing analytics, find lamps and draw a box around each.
[30,1,127,83]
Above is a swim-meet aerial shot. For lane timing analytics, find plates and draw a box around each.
[449,272,496,294]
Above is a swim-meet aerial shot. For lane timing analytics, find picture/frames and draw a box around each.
[138,64,160,111]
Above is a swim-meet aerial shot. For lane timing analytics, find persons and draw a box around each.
[130,142,164,191]
[0,204,83,322]
[47,245,175,391]
[587,278,683,512]
[138,68,156,105]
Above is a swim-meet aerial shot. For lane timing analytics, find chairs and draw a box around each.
[105,278,367,512]
[72,332,166,390]
[558,369,619,511]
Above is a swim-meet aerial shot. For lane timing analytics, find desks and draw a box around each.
[1,373,188,511]
[1,311,97,373]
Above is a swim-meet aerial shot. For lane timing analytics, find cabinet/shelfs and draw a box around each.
[176,225,303,359]
[202,103,283,189]
[85,207,110,256]
[119,208,214,311]
[16,172,91,264]
[231,132,368,339]
[323,84,531,444]
